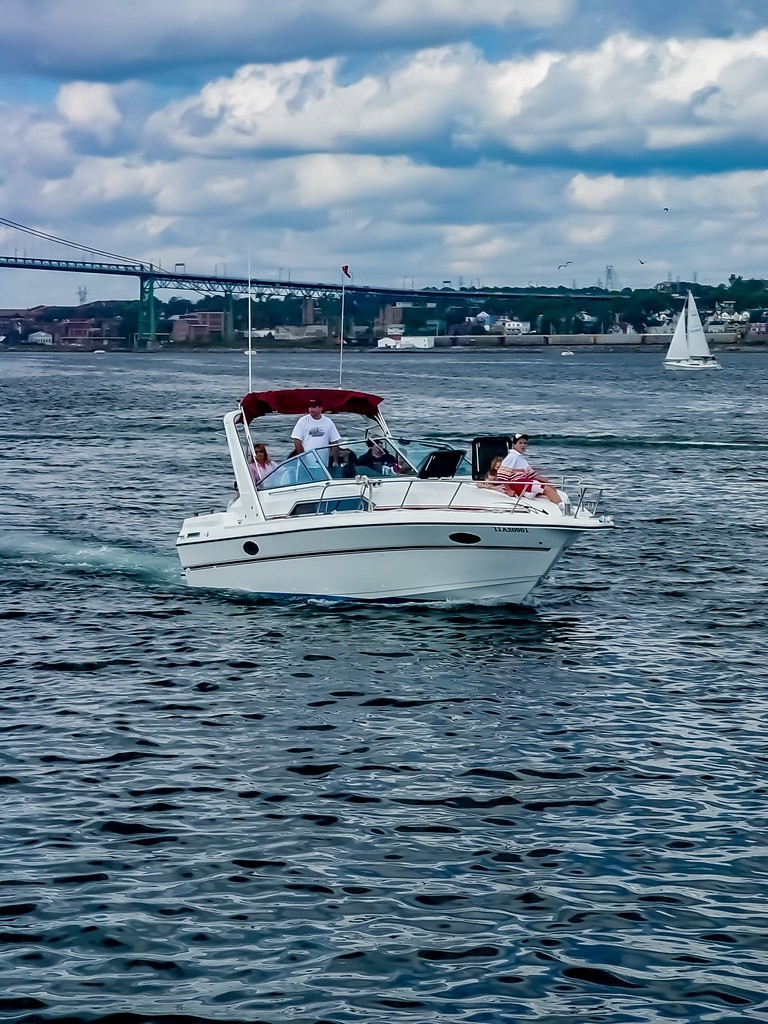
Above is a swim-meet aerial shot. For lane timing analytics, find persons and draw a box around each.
[476,433,563,508]
[286,399,412,474]
[248,444,278,483]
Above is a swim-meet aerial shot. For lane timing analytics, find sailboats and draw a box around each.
[661,288,724,371]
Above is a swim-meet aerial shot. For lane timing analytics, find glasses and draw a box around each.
[254,450,265,454]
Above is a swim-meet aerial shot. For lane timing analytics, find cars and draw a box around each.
[244,350,257,355]
[94,350,106,354]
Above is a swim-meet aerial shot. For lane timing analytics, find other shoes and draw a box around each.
[559,503,574,515]
[523,491,536,500]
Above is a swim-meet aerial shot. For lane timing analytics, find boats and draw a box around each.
[175,388,615,606]
[561,352,575,356]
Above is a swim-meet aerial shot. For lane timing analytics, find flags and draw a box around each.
[341,263,351,279]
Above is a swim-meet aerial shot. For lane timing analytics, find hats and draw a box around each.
[306,399,322,408]
[513,433,529,444]
